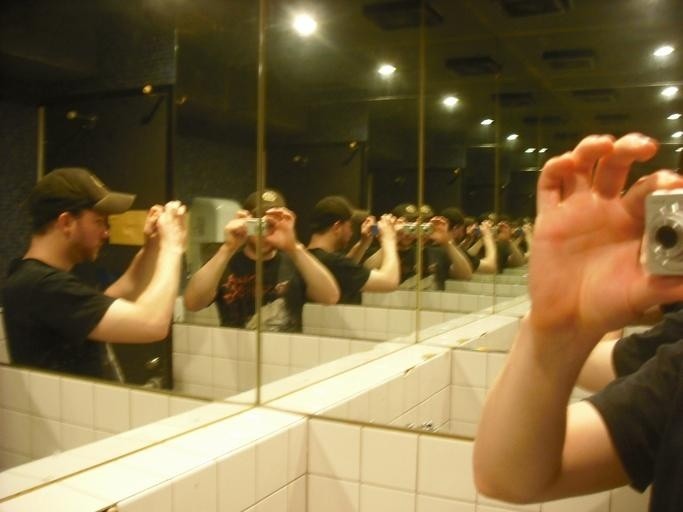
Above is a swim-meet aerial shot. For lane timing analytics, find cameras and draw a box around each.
[367,218,531,236]
[640,190,683,275]
[247,218,269,235]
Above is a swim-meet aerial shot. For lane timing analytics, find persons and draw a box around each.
[182,188,342,334]
[574,303,682,392]
[473,131,682,512]
[0,167,187,388]
[305,193,534,305]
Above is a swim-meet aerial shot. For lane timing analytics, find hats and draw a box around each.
[244,189,285,218]
[392,204,433,220]
[310,196,368,231]
[27,168,136,218]
[441,208,475,227]
